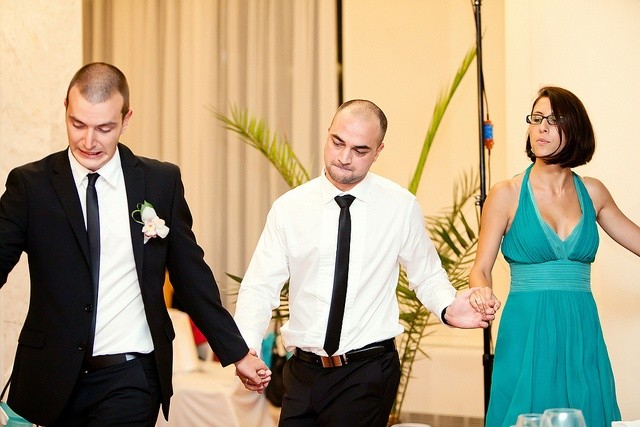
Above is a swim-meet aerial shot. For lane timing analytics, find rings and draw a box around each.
[245,378,248,383]
[475,297,481,301]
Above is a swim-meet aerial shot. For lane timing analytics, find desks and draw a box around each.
[152,358,280,427]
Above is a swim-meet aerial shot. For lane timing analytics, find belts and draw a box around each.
[293,337,397,368]
[90,352,138,370]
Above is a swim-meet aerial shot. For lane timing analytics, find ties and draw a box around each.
[324,194,357,356]
[86,172,100,373]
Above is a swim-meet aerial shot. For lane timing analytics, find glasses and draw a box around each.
[526,114,560,125]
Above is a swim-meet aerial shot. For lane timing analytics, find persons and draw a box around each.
[0,61,270,427]
[468,87,639,427]
[233,99,495,427]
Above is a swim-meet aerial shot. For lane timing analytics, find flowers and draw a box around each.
[130,199,170,245]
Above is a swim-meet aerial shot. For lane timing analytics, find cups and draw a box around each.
[515,407,586,427]
